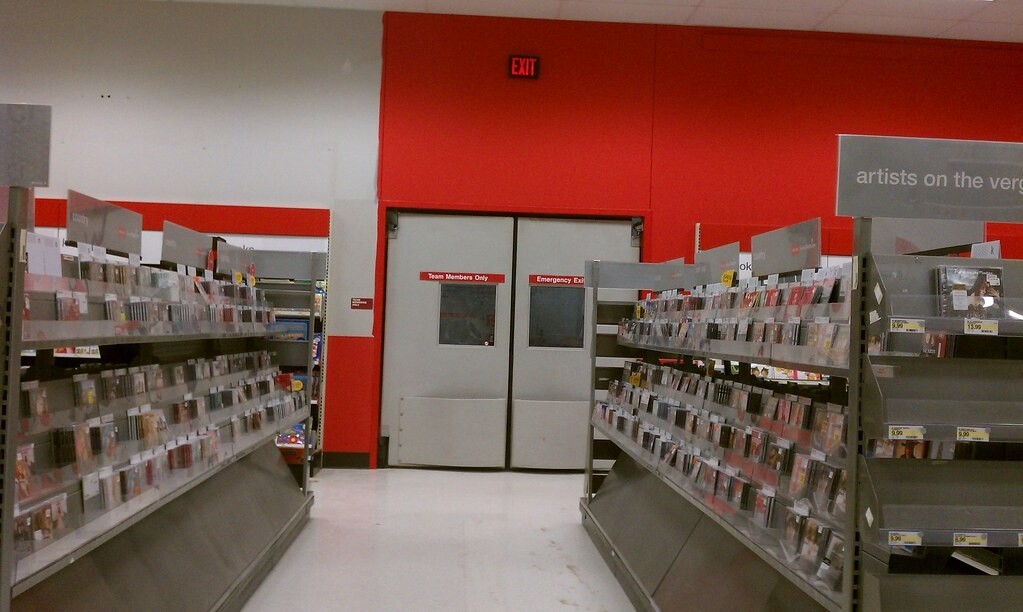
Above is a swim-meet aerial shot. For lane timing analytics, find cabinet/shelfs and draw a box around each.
[0,103,328,612]
[259,249,328,477]
[578,135,1023,612]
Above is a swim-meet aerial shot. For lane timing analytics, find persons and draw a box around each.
[900,438,919,458]
[967,269,1000,319]
